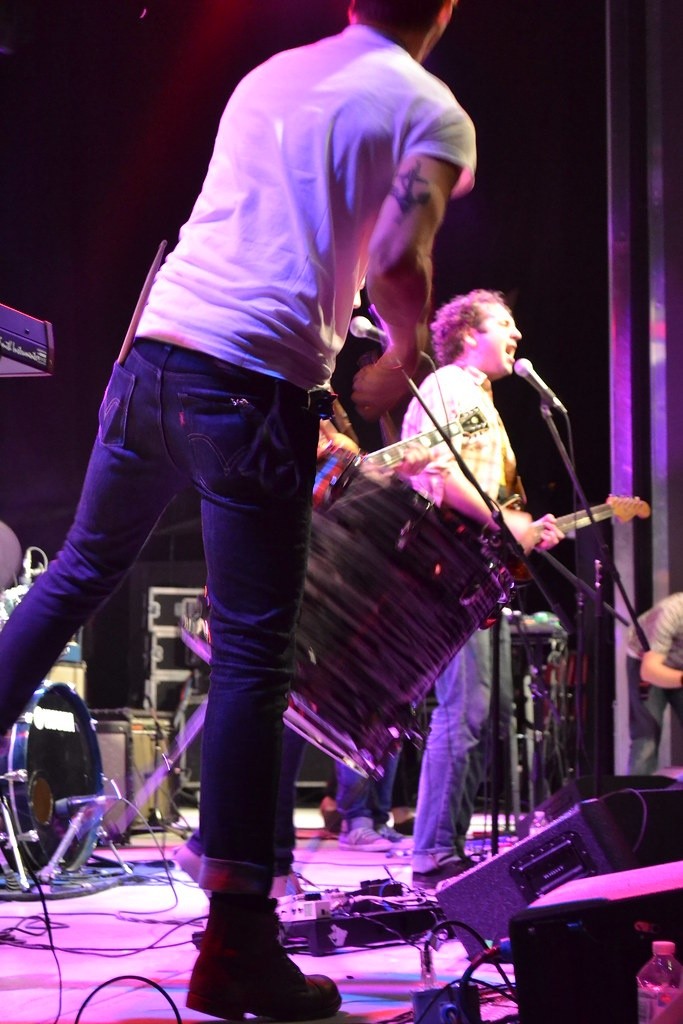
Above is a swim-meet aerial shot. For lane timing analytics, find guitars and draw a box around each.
[529,492,654,546]
[363,405,490,474]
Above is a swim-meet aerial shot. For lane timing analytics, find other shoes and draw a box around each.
[413,858,479,889]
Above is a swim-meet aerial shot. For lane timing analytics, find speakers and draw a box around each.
[435,775,683,1024]
[93,719,179,830]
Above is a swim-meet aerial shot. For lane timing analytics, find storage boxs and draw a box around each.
[143,586,333,807]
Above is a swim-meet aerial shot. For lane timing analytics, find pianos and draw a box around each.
[0,301,56,379]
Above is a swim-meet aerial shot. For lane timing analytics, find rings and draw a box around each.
[533,531,539,537]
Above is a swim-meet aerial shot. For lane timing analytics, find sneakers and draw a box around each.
[186,898,342,1022]
[339,826,414,851]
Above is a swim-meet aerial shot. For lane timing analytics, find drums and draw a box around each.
[5,582,83,665]
[178,435,531,786]
[1,676,106,875]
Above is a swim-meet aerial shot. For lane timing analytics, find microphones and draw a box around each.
[146,697,166,740]
[54,794,118,818]
[513,358,568,415]
[350,316,431,361]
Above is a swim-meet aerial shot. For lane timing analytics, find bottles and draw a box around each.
[635,940,683,1024]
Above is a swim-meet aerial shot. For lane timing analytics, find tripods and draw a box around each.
[129,735,197,840]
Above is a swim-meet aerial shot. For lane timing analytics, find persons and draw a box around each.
[171,265,365,901]
[620,586,683,781]
[0,2,489,1024]
[325,734,403,848]
[402,286,565,897]
